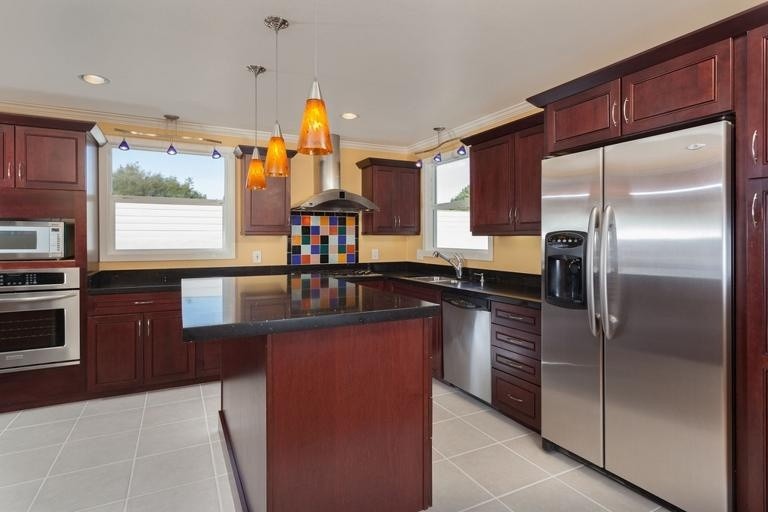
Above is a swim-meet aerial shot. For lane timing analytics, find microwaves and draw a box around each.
[0,220,66,260]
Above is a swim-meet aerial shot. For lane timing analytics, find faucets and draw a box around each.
[431,249,485,287]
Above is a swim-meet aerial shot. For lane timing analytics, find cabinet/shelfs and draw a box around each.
[733,0,768,512]
[460,110,543,235]
[359,280,443,382]
[234,144,298,235]
[357,157,420,235]
[490,302,541,434]
[527,34,734,155]
[219,317,434,511]
[0,111,107,191]
[87,291,195,396]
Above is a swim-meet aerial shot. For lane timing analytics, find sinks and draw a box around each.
[415,276,472,288]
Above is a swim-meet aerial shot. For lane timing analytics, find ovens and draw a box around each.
[0,267,81,373]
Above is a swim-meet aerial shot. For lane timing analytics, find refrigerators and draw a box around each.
[540,120,735,510]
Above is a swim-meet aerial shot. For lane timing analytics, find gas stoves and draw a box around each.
[317,268,384,278]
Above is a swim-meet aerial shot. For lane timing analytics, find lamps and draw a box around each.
[413,128,467,169]
[264,16,290,178]
[299,0,334,156]
[115,116,223,158]
[245,64,268,190]
